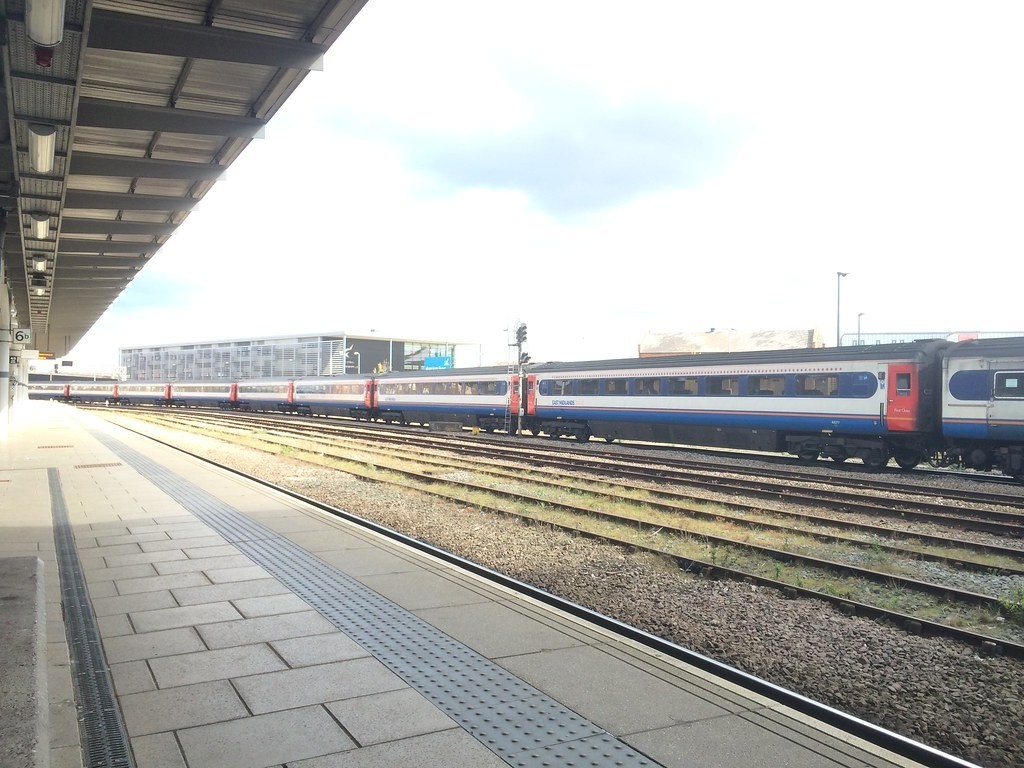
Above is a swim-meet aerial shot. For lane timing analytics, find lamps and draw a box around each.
[30,214,49,239]
[28,123,57,174]
[31,279,48,296]
[32,254,47,272]
[24,0,65,48]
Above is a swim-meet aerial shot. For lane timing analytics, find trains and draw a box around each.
[27,336,1024,482]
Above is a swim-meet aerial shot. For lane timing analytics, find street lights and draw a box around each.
[858,313,864,345]
[837,272,849,347]
